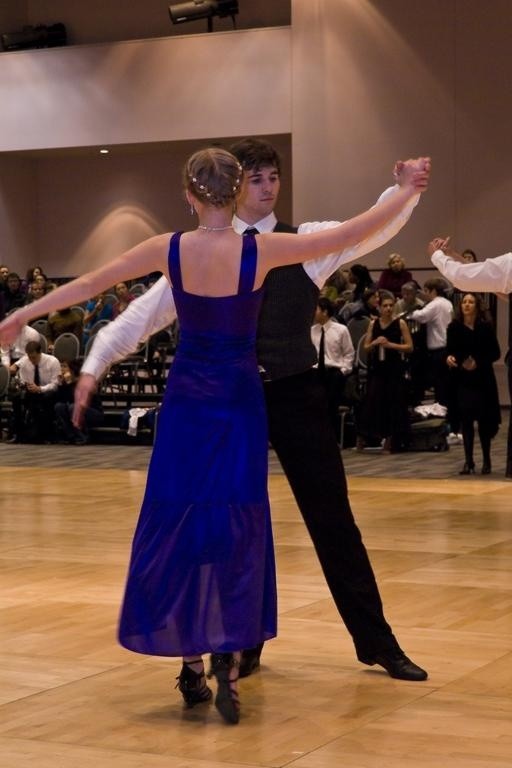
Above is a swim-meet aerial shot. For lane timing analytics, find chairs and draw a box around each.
[0,283,148,398]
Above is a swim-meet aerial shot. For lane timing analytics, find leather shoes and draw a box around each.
[357,652,427,681]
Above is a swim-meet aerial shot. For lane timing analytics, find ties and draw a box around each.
[319,327,324,365]
[34,364,40,385]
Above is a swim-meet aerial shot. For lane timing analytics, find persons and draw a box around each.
[310,236,512,481]
[71,137,431,682]
[0,265,163,446]
[0,147,426,725]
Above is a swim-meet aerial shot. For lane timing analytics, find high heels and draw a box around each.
[482,462,492,474]
[459,462,476,474]
[174,642,264,724]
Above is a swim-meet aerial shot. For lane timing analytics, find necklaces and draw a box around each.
[198,225,234,231]
